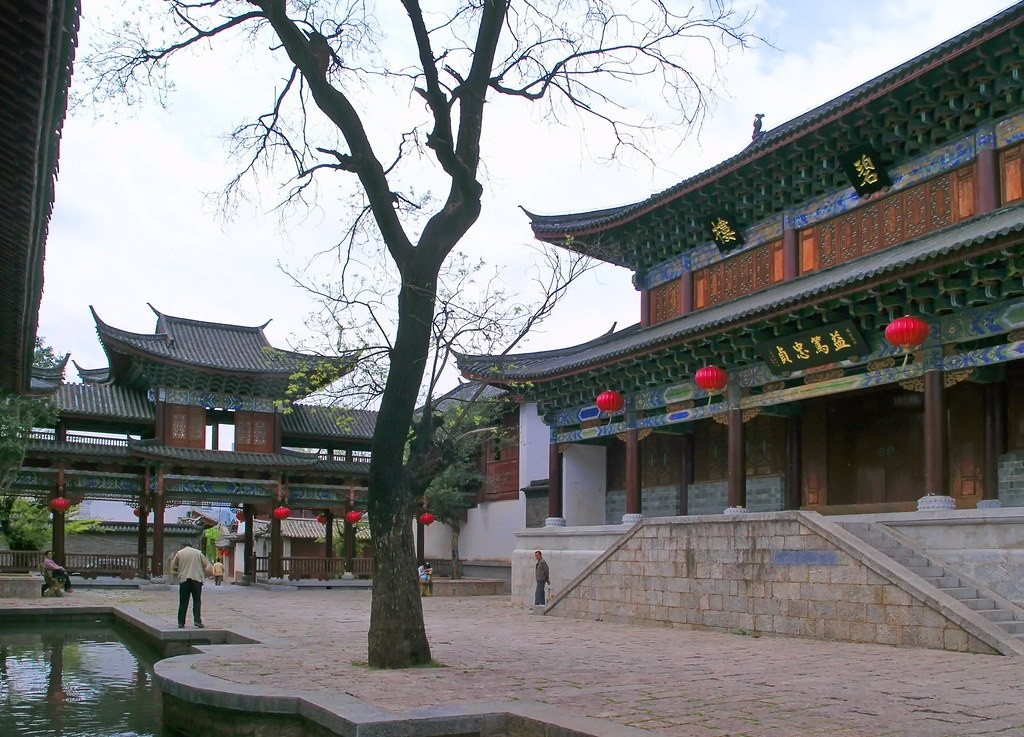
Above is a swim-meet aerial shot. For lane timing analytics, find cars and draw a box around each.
[80,559,136,579]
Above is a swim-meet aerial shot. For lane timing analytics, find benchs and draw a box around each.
[39,563,65,597]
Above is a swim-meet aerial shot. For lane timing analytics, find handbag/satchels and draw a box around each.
[419,573,431,585]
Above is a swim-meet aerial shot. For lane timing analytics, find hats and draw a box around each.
[423,560,432,568]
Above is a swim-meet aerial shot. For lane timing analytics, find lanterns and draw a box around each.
[885,315,929,367]
[420,513,434,526]
[317,514,326,524]
[52,497,70,511]
[597,390,624,422]
[134,509,149,517]
[236,511,246,523]
[696,365,728,406]
[347,511,361,527]
[274,506,291,520]
[224,550,230,556]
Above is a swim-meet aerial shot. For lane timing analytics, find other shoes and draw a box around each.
[177,622,184,628]
[194,620,204,627]
[66,589,74,593]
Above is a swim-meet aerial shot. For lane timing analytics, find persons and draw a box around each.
[172,540,207,627]
[45,551,73,593]
[535,551,550,605]
[213,559,224,585]
[418,562,433,596]
